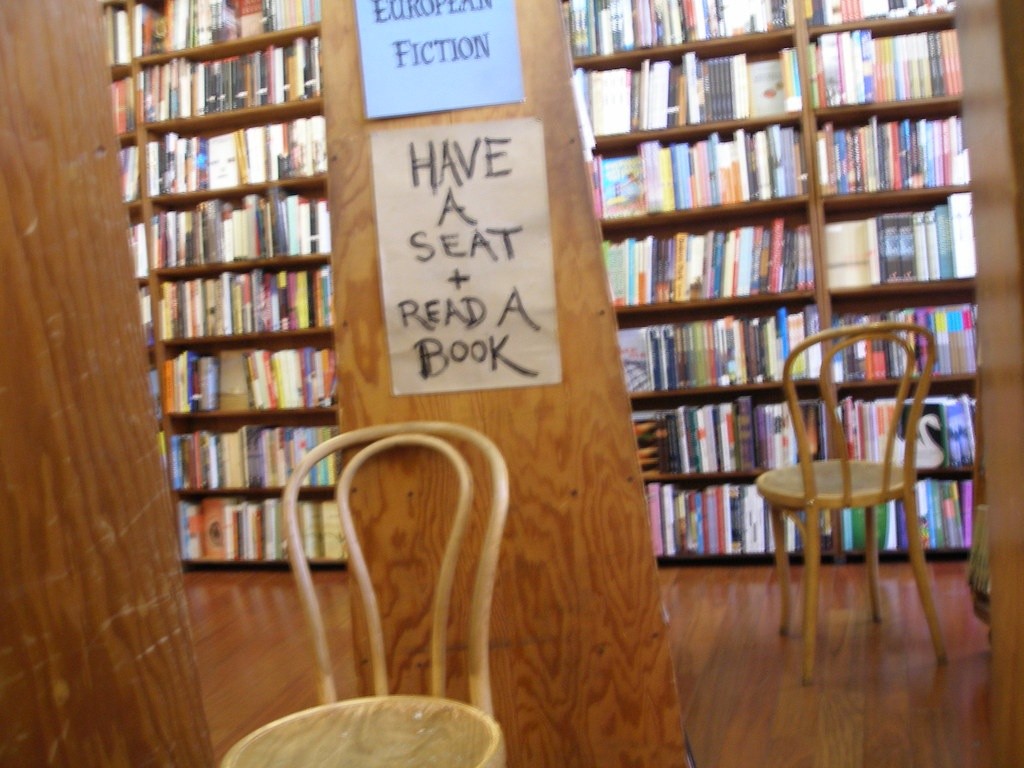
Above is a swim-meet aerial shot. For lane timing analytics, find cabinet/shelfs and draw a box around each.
[100,0,976,571]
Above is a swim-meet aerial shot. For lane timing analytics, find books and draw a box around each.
[103,0,981,564]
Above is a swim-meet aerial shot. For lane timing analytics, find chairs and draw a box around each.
[755,322,952,690]
[220,419,512,768]
